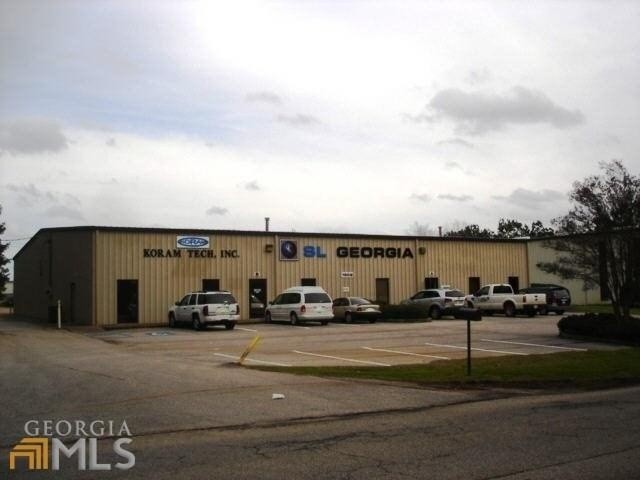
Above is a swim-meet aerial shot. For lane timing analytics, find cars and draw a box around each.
[127,292,137,321]
[332,296,383,324]
[251,294,264,315]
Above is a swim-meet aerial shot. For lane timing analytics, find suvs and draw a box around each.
[166,288,242,332]
[399,287,467,321]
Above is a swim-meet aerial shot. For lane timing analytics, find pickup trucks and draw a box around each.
[463,282,547,318]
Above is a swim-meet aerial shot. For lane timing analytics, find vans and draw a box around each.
[263,285,336,328]
[512,285,572,317]
